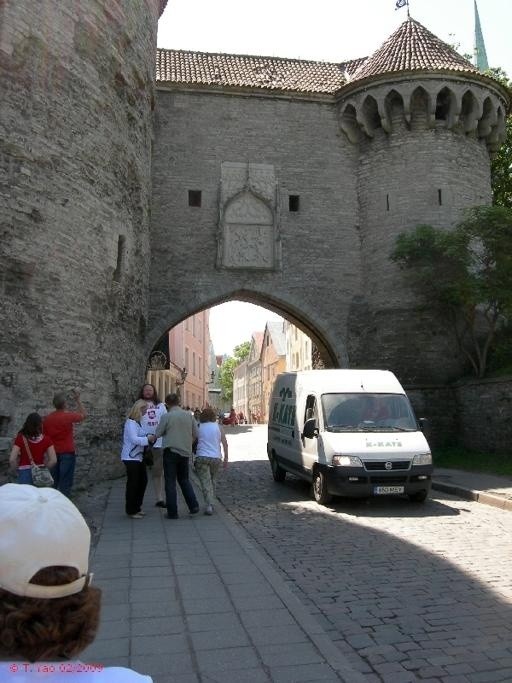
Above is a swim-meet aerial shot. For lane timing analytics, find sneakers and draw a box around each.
[204,505,213,514]
[138,509,146,514]
[131,513,143,518]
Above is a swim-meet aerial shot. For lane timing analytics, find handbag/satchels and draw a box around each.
[32,463,55,486]
[129,432,157,465]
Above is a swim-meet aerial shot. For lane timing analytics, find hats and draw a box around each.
[0,482,94,599]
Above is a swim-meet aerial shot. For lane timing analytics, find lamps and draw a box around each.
[177,367,188,385]
[206,370,216,384]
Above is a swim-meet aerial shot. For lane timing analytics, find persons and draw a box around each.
[2,480,155,683]
[43,386,90,493]
[121,381,247,520]
[10,412,57,485]
[361,396,392,421]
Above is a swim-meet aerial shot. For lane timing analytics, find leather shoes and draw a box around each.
[165,513,178,518]
[156,500,166,508]
[189,506,199,513]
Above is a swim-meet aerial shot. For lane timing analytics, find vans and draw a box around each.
[264,366,434,507]
